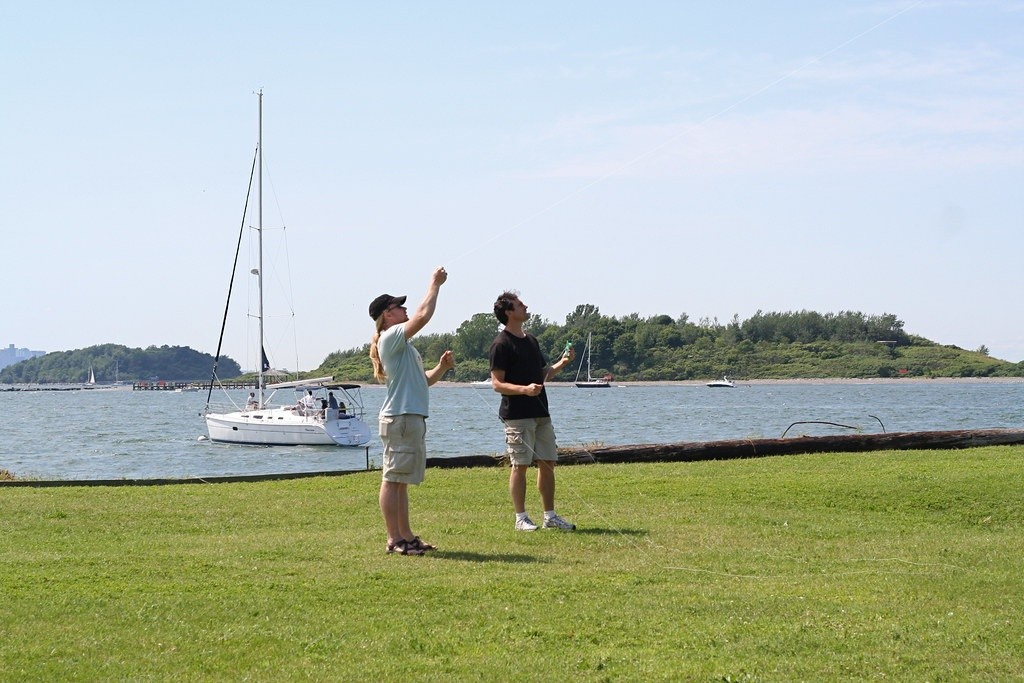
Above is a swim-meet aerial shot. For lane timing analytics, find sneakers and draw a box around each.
[542,512,576,531]
[515,516,539,531]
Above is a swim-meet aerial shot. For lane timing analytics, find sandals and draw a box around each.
[385,539,425,555]
[410,536,438,551]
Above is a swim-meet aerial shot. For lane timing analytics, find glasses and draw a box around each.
[388,302,401,312]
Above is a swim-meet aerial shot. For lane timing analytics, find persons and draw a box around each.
[247,393,258,410]
[489,292,577,531]
[328,392,338,409]
[301,391,315,408]
[369,267,455,556]
[339,402,346,414]
[321,397,328,418]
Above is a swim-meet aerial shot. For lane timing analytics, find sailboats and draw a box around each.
[82,365,96,386]
[575,332,611,388]
[200,86,373,446]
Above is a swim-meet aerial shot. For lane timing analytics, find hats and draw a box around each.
[369,294,406,321]
[328,392,333,395]
[339,402,344,405]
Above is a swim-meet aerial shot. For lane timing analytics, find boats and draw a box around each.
[706,375,735,387]
[175,384,201,393]
